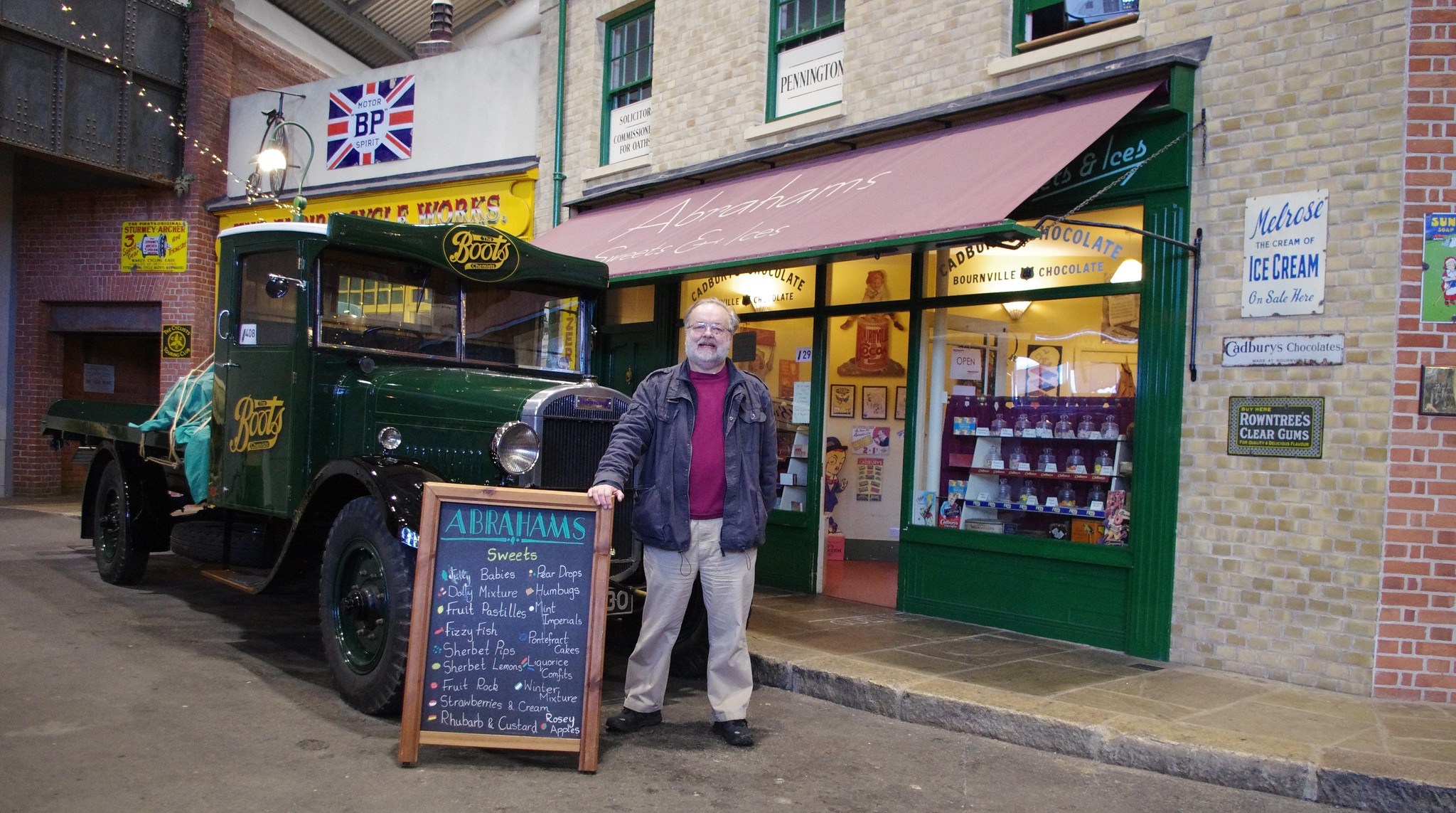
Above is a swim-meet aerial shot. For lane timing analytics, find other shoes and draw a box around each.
[605,707,662,730]
[712,719,754,745]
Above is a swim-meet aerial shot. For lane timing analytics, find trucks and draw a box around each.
[43,208,711,717]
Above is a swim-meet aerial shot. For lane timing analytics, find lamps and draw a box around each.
[257,124,288,172]
[1003,300,1030,320]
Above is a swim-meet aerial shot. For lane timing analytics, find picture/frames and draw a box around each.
[861,385,887,419]
[830,383,855,419]
[895,385,907,420]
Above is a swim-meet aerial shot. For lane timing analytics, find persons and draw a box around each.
[587,297,781,749]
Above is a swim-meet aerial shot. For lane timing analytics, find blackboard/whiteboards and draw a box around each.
[399,480,616,754]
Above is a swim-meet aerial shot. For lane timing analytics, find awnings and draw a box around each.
[523,77,1182,288]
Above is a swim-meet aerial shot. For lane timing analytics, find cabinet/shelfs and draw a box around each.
[938,394,1136,542]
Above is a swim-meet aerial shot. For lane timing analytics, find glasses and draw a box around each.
[687,323,731,334]
